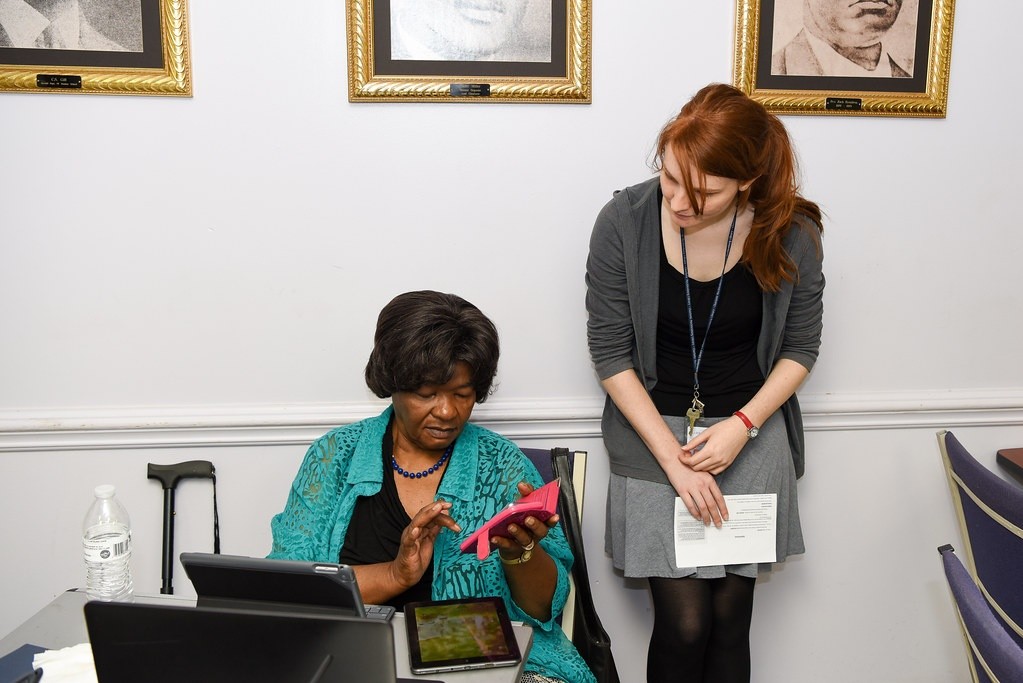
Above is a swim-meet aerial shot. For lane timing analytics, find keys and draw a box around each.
[687,407,699,436]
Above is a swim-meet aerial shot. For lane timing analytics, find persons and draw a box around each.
[586,83,826,683]
[260,290,599,683]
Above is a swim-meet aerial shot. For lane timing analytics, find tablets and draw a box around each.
[404,596,522,674]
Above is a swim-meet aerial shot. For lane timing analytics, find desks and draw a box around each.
[0,587,534,683]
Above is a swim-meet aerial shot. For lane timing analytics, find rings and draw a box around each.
[522,540,535,550]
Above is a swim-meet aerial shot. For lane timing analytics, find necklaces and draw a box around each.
[392,444,453,478]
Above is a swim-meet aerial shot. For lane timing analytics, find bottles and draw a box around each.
[80,483,131,598]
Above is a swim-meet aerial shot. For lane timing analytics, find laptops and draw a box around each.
[178,551,397,622]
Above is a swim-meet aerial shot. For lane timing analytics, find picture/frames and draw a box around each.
[0,0,193,99]
[734,0,955,119]
[346,0,593,106]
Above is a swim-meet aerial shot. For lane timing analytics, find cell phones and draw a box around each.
[461,500,543,557]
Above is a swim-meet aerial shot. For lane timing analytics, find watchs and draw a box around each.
[497,549,533,565]
[734,411,759,439]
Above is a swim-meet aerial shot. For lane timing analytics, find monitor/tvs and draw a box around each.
[85,599,397,683]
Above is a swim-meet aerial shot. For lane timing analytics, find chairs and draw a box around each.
[519,445,587,646]
[924,427,1023,683]
